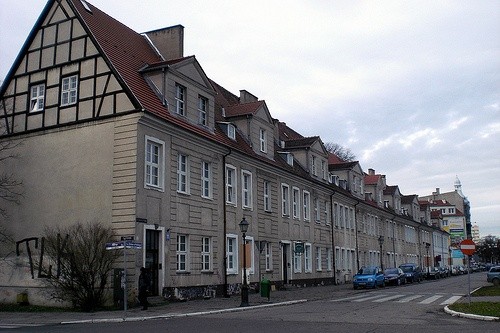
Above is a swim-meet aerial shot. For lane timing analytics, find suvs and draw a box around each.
[400,263,422,283]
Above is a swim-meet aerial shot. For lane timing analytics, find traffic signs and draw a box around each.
[125,242,141,249]
[105,241,125,250]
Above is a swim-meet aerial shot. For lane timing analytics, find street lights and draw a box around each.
[239,217,249,306]
[425,244,430,273]
[378,235,384,270]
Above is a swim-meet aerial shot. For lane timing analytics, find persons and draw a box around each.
[138,267,150,310]
[113,273,124,309]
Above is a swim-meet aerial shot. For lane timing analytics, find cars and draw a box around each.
[487,266,500,284]
[383,268,407,286]
[423,263,485,280]
[353,265,385,289]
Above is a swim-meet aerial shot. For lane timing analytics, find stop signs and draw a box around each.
[460,239,476,256]
